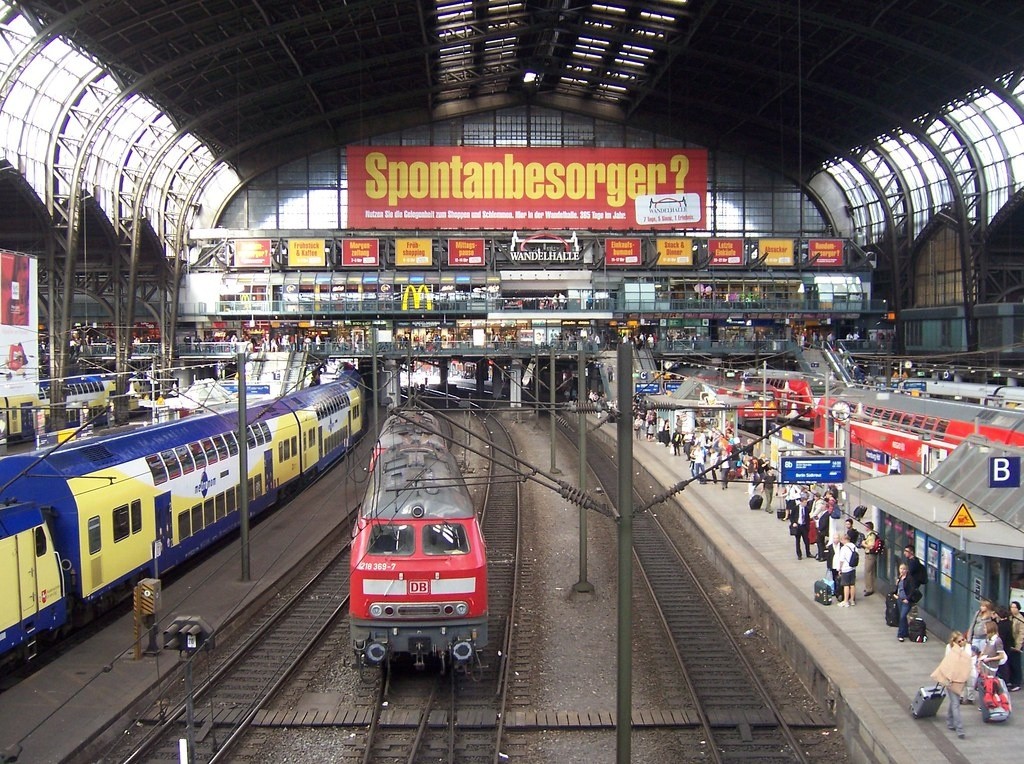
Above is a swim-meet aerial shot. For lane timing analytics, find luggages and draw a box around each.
[909,617,927,642]
[749,492,764,509]
[853,505,867,521]
[978,658,1012,723]
[909,681,946,719]
[808,517,817,544]
[777,498,785,518]
[885,582,900,627]
[822,568,835,596]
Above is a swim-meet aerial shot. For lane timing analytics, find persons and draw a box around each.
[959,646,979,704]
[607,364,615,382]
[991,605,1013,692]
[886,453,900,475]
[978,621,1005,710]
[564,386,877,607]
[894,561,915,642]
[853,366,860,383]
[944,630,972,737]
[903,545,920,635]
[1006,601,1024,692]
[966,598,999,691]
[398,293,895,353]
[39,332,362,355]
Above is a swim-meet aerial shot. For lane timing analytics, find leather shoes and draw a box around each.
[807,553,815,558]
[798,555,802,560]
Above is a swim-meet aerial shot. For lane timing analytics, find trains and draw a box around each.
[812,386,1024,476]
[666,362,839,435]
[349,404,491,684]
[0,359,362,674]
[0,371,173,446]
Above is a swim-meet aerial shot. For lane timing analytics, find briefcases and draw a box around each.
[835,576,850,601]
[814,580,832,605]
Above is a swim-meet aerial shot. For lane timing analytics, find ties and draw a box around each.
[802,507,805,525]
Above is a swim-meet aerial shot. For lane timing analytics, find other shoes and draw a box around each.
[863,589,874,596]
[898,637,904,642]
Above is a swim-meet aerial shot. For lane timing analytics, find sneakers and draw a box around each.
[851,601,856,605]
[1010,686,1021,691]
[837,602,849,608]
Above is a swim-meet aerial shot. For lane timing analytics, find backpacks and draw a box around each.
[830,500,840,519]
[852,528,865,549]
[867,531,885,555]
[844,545,859,567]
[919,563,929,584]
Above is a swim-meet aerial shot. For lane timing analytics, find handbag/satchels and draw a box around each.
[910,585,923,603]
[789,523,798,535]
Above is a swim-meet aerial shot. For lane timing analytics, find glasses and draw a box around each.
[958,638,964,643]
